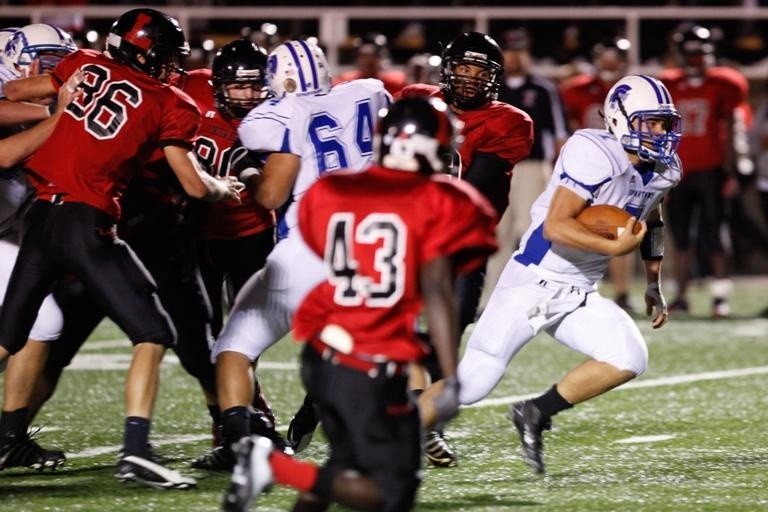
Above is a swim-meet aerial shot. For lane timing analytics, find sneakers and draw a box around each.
[0,421,67,471]
[191,391,459,512]
[114,445,198,490]
[509,400,552,474]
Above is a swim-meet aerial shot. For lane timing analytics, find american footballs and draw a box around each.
[576,204,641,241]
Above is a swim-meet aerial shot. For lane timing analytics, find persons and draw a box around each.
[328,23,768,319]
[0,6,292,491]
[227,95,499,511]
[391,32,532,469]
[414,74,682,472]
[210,39,394,511]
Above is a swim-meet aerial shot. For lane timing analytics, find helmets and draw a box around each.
[105,8,191,84]
[378,83,462,173]
[439,31,504,112]
[673,24,713,63]
[603,74,682,172]
[212,40,267,119]
[3,22,78,78]
[266,40,329,97]
[0,27,20,65]
